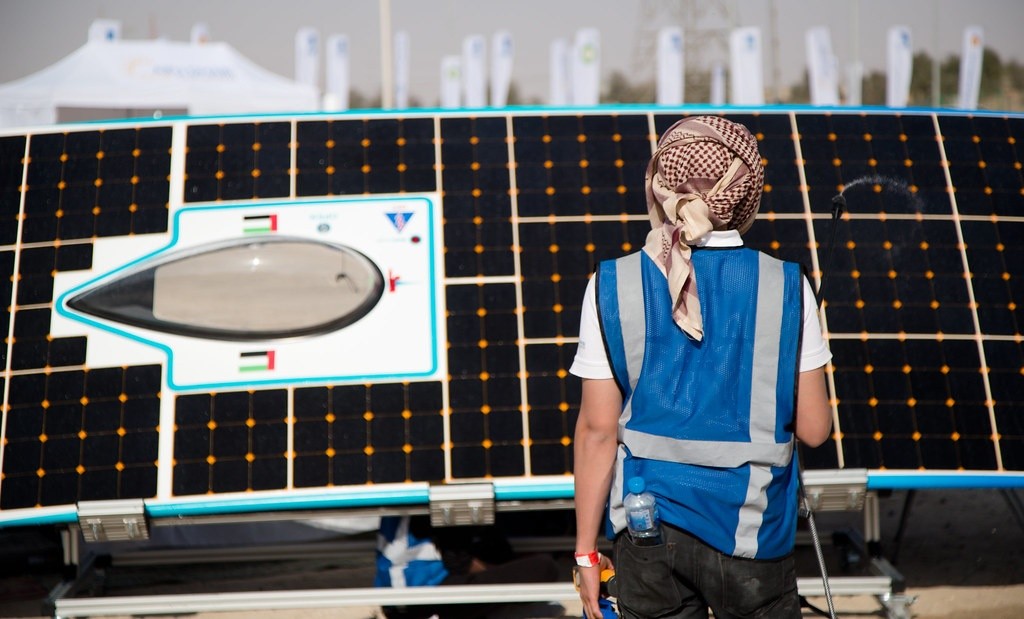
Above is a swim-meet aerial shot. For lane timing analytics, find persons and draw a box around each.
[374,516,561,619]
[567,114,833,619]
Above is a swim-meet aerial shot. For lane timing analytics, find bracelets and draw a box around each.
[574,549,601,568]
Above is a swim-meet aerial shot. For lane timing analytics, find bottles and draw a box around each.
[623,477,661,546]
